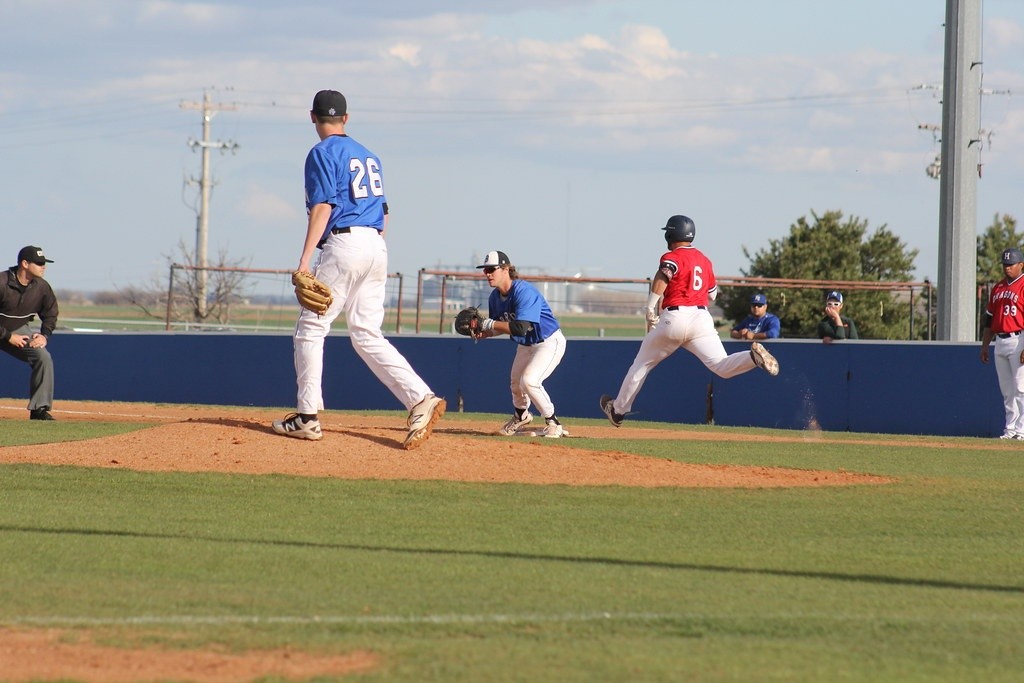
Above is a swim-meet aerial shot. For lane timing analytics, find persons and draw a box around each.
[818,291,859,344]
[272,89,447,451]
[454,251,566,440]
[981,249,1024,440]
[0,246,58,420]
[599,215,778,428]
[730,294,780,340]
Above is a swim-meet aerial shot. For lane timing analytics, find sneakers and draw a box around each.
[751,342,779,376]
[403,394,446,451]
[272,414,322,441]
[499,413,533,436]
[543,420,564,439]
[30,407,56,420]
[600,394,626,427]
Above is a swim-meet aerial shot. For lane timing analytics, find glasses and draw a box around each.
[483,266,502,274]
[35,261,45,265]
[751,303,764,307]
[827,302,841,307]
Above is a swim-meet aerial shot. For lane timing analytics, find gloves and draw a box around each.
[646,311,660,333]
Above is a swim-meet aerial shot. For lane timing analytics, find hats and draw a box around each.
[827,291,843,304]
[313,89,348,116]
[19,246,54,264]
[998,249,1024,265]
[752,293,767,304]
[476,251,510,269]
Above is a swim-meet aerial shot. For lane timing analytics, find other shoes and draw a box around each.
[999,431,1024,440]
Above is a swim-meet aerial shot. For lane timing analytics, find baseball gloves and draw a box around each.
[454,304,485,336]
[291,270,333,316]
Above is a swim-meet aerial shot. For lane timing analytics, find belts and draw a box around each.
[667,305,706,310]
[997,331,1021,339]
[332,226,381,235]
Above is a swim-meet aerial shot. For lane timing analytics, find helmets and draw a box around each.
[661,215,695,249]
